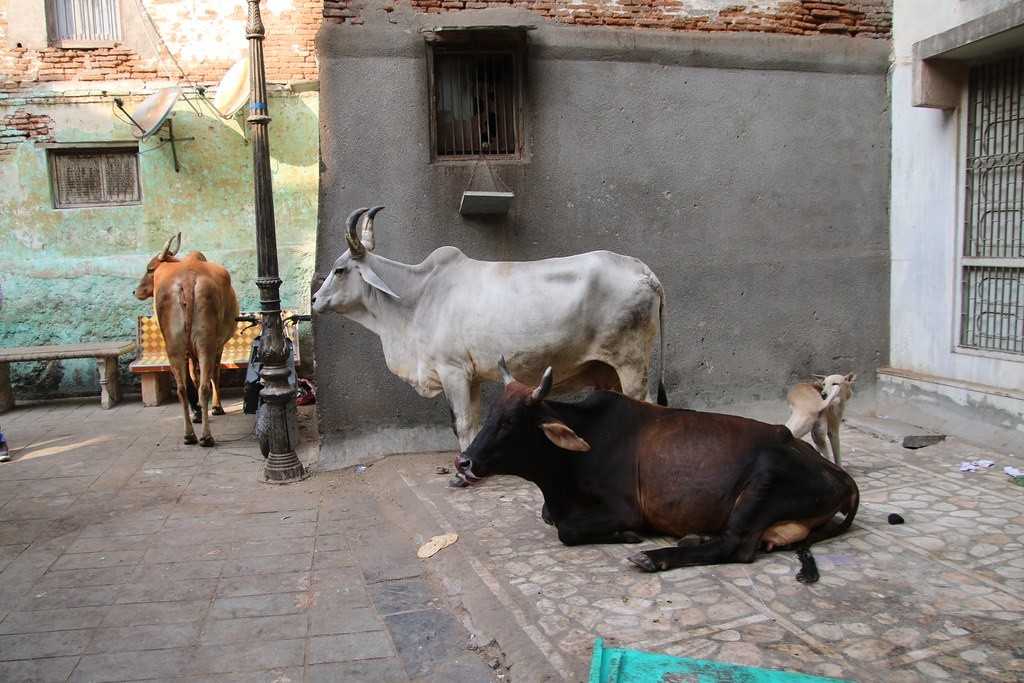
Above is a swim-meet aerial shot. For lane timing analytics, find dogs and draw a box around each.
[785,372,857,468]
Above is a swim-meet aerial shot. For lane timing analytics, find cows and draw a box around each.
[312,206,667,488]
[133,232,239,447]
[455,355,859,584]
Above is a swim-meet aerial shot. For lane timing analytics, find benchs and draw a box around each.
[129,310,301,407]
[0,340,137,413]
